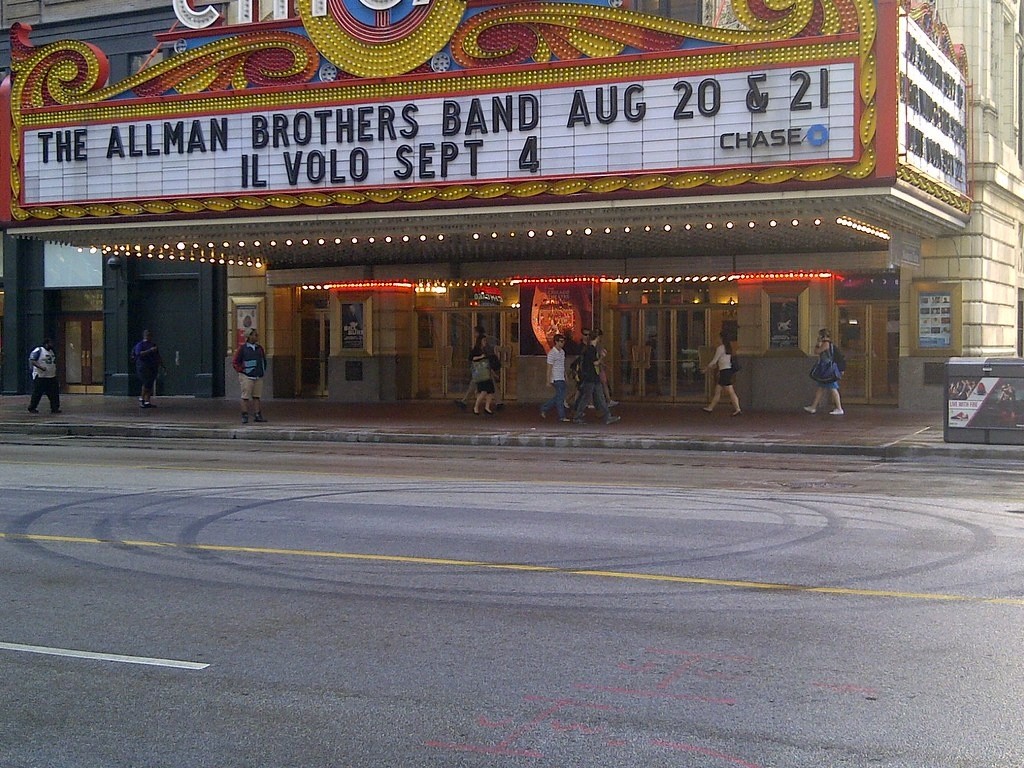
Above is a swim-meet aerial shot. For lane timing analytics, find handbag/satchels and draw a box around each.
[809,340,841,384]
[731,354,739,371]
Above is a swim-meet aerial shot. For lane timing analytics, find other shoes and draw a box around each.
[560,418,570,422]
[829,409,844,415]
[804,406,816,414]
[608,400,619,407]
[606,416,621,425]
[496,403,506,408]
[702,408,712,413]
[140,400,156,408]
[455,400,466,409]
[731,410,742,416]
[28,408,39,414]
[51,410,62,413]
[563,400,570,408]
[539,411,546,419]
[572,418,587,424]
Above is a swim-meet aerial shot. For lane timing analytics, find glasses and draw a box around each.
[582,333,589,336]
[556,340,565,343]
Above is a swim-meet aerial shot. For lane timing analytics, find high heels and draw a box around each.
[473,408,479,416]
[484,408,494,415]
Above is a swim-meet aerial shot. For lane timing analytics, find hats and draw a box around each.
[244,328,255,337]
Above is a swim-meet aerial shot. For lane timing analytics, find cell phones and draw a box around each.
[821,334,825,339]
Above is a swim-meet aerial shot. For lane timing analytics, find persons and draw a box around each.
[700,332,742,417]
[135,329,161,408]
[564,328,622,425]
[27,337,62,414]
[539,334,570,422]
[233,328,268,423]
[803,329,844,415]
[456,327,502,416]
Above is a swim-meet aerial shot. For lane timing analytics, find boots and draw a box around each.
[241,411,248,423]
[254,412,267,422]
